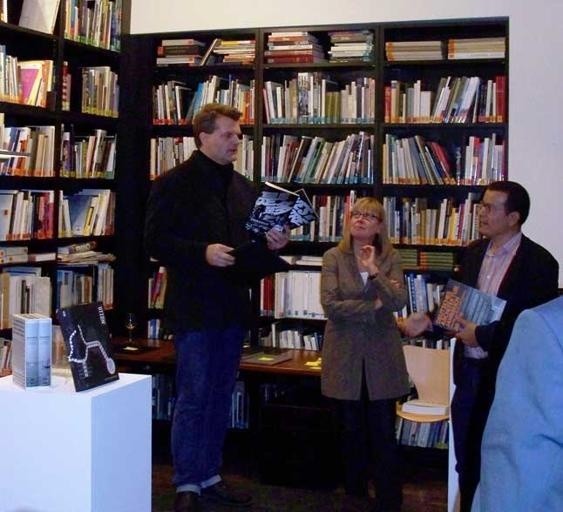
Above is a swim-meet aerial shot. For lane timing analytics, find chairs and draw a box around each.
[394,345,449,482]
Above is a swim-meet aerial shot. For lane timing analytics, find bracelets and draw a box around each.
[366,273,379,280]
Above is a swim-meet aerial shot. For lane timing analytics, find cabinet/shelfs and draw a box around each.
[135,17,508,354]
[1,0,131,378]
[1,369,153,507]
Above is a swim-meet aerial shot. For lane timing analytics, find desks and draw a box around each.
[108,338,325,429]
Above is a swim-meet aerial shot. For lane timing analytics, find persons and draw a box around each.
[320,197,409,512]
[468,295,563,512]
[144,103,291,512]
[397,181,559,512]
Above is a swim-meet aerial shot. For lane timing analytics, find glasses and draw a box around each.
[479,201,505,214]
[352,212,381,221]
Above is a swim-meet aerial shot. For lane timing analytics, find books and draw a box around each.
[64,0,122,53]
[0,0,61,35]
[58,188,116,239]
[264,32,328,64]
[56,300,120,392]
[200,39,256,66]
[61,61,120,119]
[279,255,325,268]
[263,71,376,125]
[259,271,328,321]
[287,188,319,241]
[151,372,177,422]
[434,277,508,334]
[385,41,447,61]
[12,313,52,392]
[447,36,506,60]
[239,351,292,366]
[245,181,299,236]
[393,415,450,451]
[262,130,375,184]
[0,189,55,241]
[400,249,458,273]
[455,130,505,185]
[0,337,12,377]
[394,273,452,348]
[327,30,375,63]
[156,38,206,67]
[56,241,116,311]
[382,133,456,185]
[230,136,254,181]
[0,113,55,178]
[0,45,59,111]
[59,124,118,179]
[320,190,400,243]
[147,267,167,339]
[230,379,285,430]
[256,321,323,351]
[148,136,198,181]
[401,192,487,246]
[384,74,506,123]
[0,245,56,330]
[401,398,449,416]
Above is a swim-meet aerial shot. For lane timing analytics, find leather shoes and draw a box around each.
[203,480,255,505]
[175,491,199,512]
